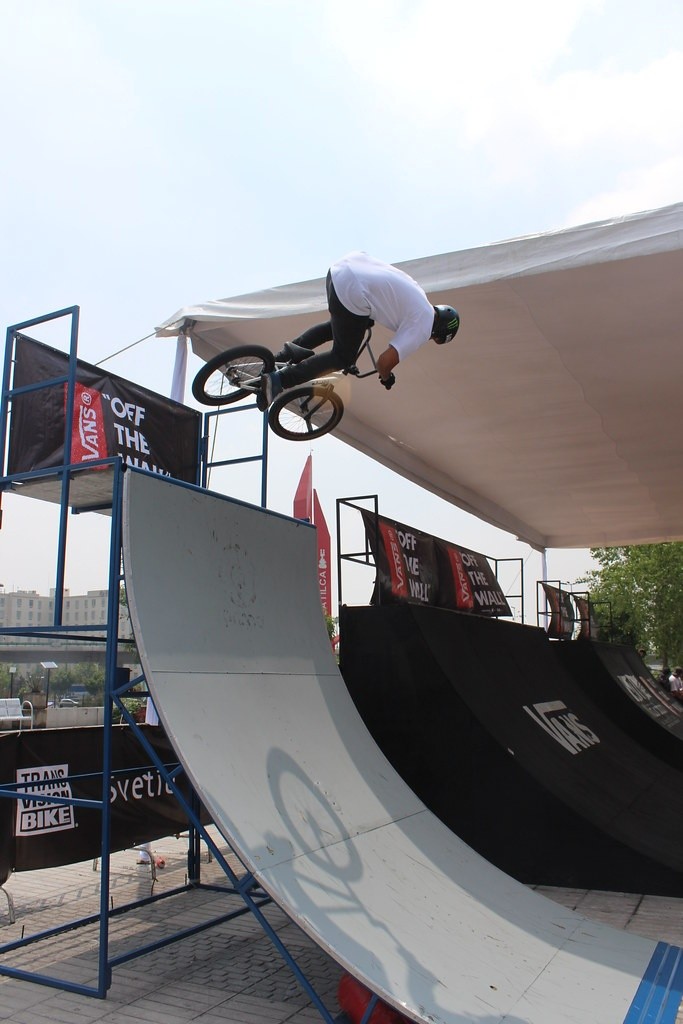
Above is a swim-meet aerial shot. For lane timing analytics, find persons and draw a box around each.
[669,668,683,691]
[639,650,647,659]
[655,667,671,691]
[256,252,458,411]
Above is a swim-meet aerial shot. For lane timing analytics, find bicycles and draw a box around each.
[191,312,389,441]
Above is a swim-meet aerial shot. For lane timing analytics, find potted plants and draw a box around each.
[22,671,47,709]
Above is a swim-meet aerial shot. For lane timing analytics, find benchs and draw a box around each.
[0,698,34,729]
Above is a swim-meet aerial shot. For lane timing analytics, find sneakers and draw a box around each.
[256,371,283,412]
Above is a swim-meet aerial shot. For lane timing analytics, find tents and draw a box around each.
[132,201,683,865]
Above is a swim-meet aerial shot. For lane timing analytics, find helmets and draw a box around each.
[430,304,460,344]
